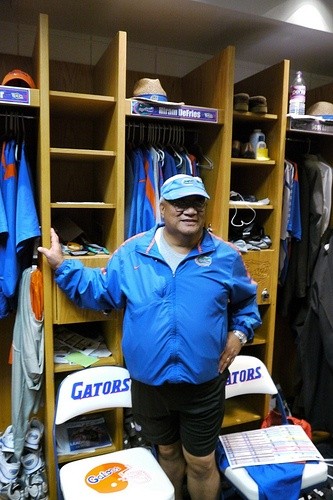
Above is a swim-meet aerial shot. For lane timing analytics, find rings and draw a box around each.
[226,358,230,363]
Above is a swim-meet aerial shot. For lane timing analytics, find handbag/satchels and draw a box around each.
[261,410,312,440]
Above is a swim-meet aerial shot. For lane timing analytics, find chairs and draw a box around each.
[215,353,329,500]
[52,366,176,500]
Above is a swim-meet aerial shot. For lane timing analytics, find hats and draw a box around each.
[2,69,36,89]
[129,78,185,105]
[160,174,210,200]
[306,101,333,121]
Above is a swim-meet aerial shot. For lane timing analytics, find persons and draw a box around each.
[37,174,261,500]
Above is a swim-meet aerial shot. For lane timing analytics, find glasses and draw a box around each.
[166,200,207,212]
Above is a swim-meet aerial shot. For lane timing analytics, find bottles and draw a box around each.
[250,129,265,156]
[256,136,266,159]
[289,71,306,116]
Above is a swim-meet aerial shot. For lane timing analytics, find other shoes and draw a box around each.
[231,141,253,158]
[233,93,268,112]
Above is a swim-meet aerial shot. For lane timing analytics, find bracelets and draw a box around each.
[233,330,246,347]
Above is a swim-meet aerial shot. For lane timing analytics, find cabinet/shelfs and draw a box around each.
[0,13,333,500]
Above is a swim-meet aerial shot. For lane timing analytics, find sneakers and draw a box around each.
[0,416,48,500]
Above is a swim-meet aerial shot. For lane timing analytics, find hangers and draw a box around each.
[3,112,27,160]
[304,137,319,158]
[126,120,214,172]
